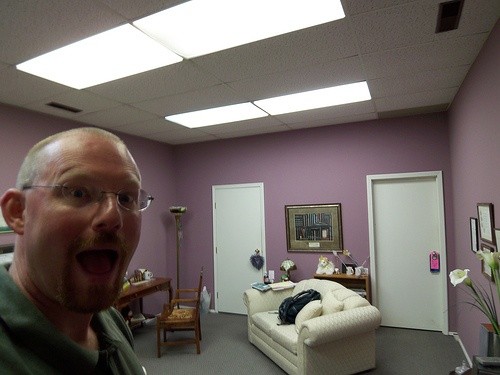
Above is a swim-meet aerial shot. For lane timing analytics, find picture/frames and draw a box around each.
[476,202,495,246]
[493,229,500,252]
[470,217,479,254]
[480,242,494,282]
[284,202,344,253]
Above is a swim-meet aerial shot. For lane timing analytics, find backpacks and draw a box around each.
[276,289,322,325]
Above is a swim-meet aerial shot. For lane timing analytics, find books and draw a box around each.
[252,281,295,292]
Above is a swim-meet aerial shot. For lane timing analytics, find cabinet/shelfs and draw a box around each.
[314,274,371,303]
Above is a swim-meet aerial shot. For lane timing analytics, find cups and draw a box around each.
[144,270,153,280]
[354,267,362,276]
[345,267,354,276]
[361,268,370,276]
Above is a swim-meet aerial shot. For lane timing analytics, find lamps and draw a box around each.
[280,257,297,281]
[168,206,186,309]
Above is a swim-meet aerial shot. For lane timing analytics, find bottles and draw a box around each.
[264,271,268,285]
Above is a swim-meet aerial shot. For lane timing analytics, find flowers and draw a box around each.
[448,247,500,334]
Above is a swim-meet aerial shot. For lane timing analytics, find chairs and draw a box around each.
[156,266,204,359]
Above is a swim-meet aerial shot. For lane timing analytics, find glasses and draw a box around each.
[22,180,156,212]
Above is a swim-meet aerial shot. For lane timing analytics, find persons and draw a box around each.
[0,127,148,375]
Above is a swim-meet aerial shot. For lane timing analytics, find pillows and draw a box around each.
[321,290,344,315]
[294,300,322,335]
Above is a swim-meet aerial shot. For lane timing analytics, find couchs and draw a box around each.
[243,278,382,375]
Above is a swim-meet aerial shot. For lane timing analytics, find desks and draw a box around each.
[115,276,172,328]
